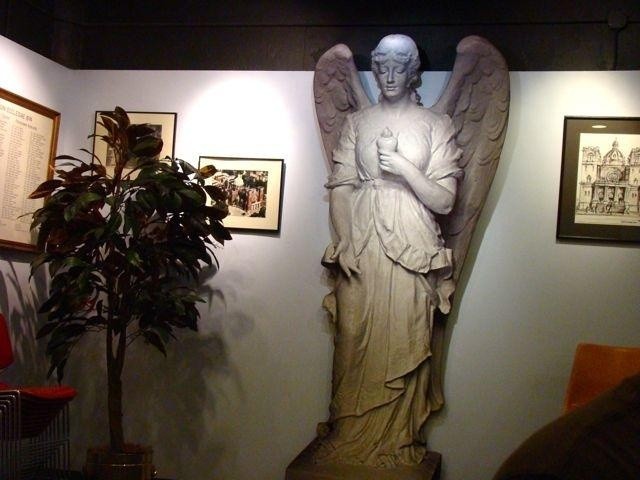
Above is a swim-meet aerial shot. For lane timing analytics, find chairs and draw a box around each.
[0,310,79,480]
[559,342,640,420]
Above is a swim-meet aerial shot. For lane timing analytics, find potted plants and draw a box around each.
[27,106,233,480]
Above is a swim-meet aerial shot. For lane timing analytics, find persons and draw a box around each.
[311,33,464,467]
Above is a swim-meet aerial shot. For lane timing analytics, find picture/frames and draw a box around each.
[198,155,283,233]
[92,110,178,184]
[556,115,640,244]
[0,87,61,255]
